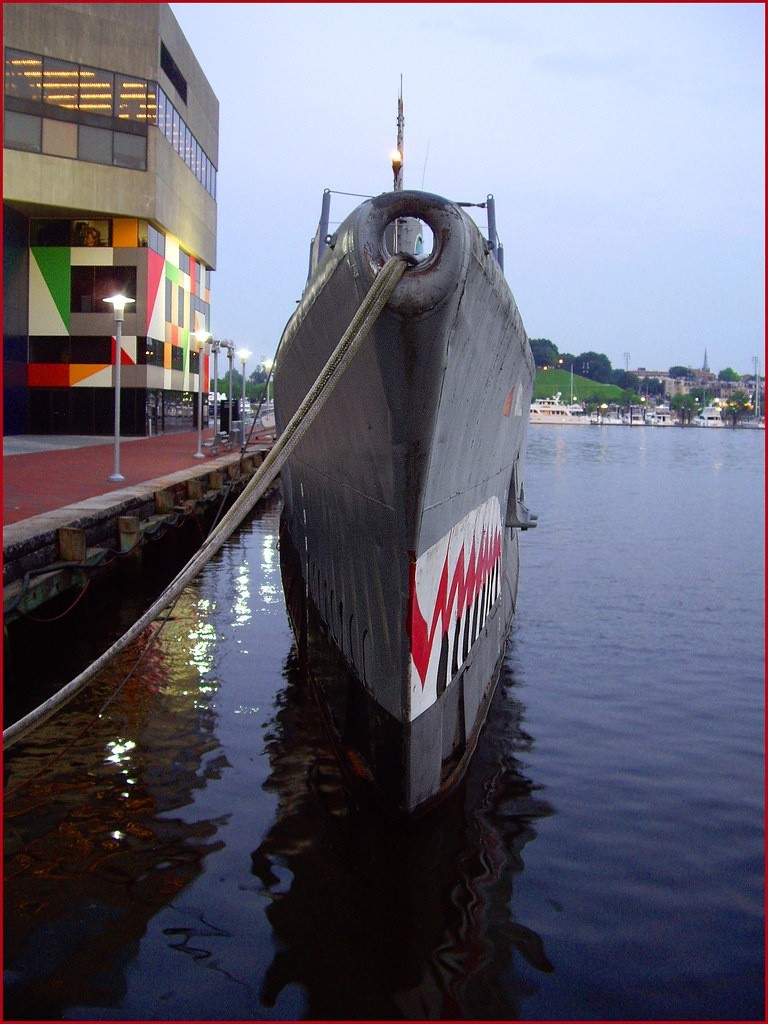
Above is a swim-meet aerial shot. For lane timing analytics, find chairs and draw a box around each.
[202,431,227,456]
[222,429,240,450]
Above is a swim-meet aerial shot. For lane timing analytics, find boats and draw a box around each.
[272,76,540,821]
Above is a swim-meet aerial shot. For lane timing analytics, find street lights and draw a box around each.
[236,349,253,448]
[262,359,276,420]
[102,293,135,482]
[191,329,212,460]
